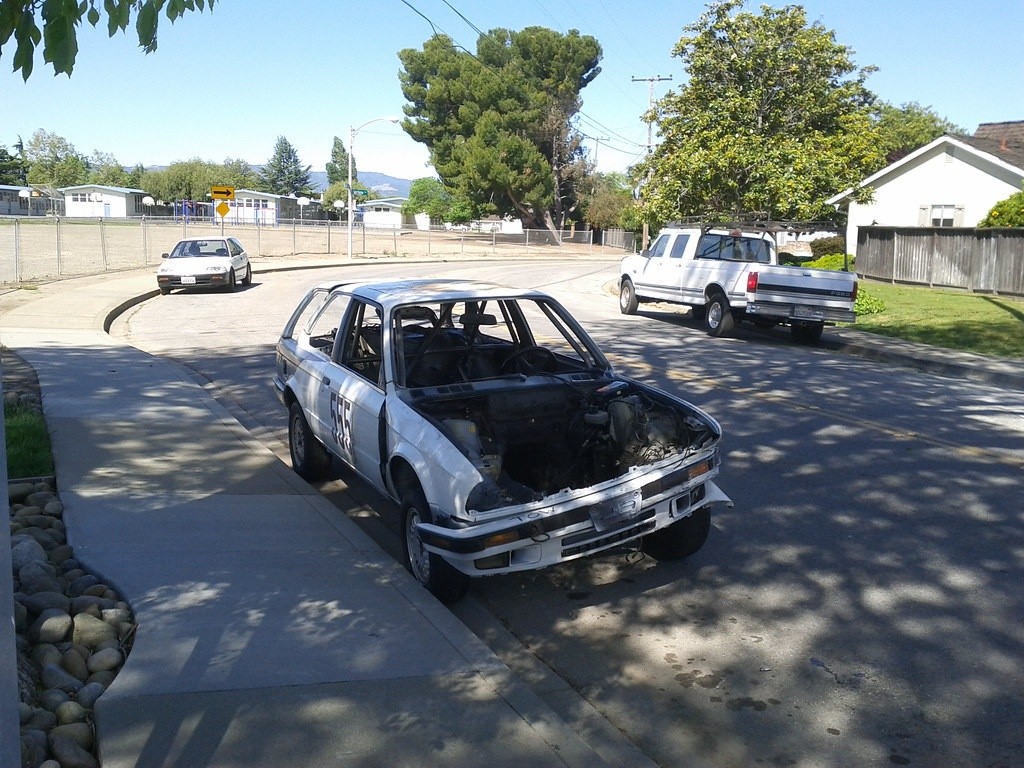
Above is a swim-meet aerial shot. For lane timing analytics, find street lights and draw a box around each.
[346,117,399,257]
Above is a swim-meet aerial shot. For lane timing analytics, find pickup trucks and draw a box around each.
[618,228,858,345]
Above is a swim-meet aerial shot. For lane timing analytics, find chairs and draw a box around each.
[216,248,226,256]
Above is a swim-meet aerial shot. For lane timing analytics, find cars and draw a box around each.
[157,236,252,295]
[274,276,735,591]
